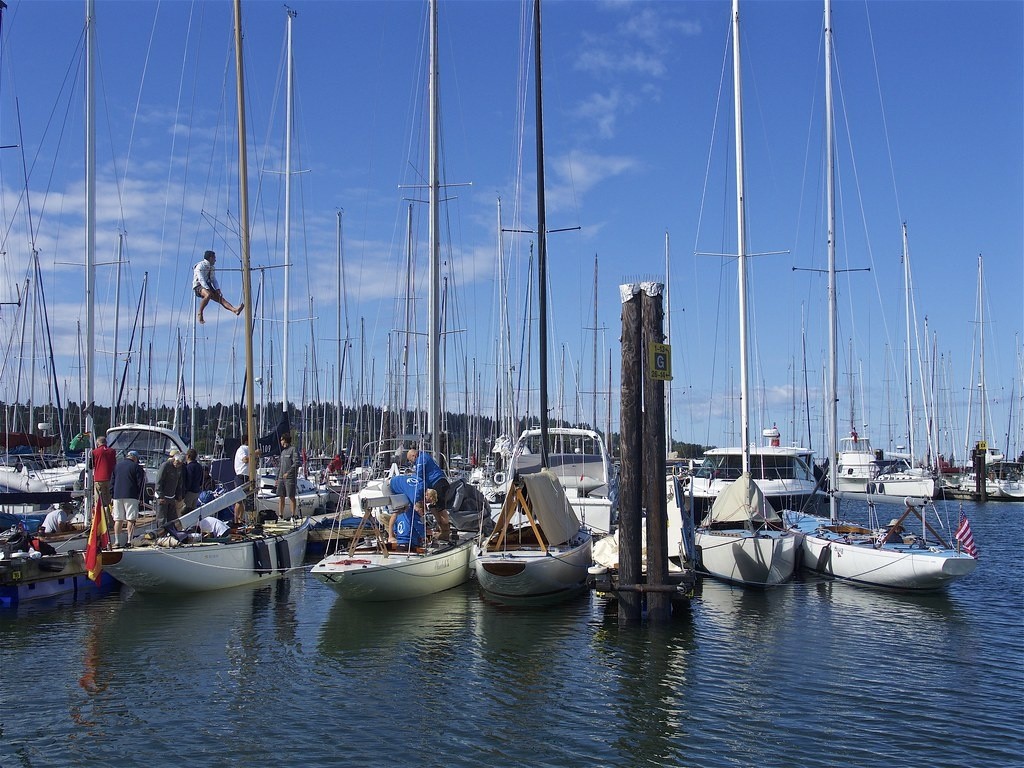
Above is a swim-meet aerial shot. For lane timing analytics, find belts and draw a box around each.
[386,477,391,489]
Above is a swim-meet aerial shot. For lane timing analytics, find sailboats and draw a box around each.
[1,2,1023,607]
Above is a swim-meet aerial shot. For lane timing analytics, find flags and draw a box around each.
[954,508,979,560]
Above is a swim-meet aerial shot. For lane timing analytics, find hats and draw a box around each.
[130,451,140,461]
[282,433,292,443]
[185,449,197,459]
[62,502,73,510]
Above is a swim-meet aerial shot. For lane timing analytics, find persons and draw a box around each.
[193,251,244,324]
[90,433,452,550]
[39,502,74,534]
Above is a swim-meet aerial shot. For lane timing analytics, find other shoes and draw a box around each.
[123,543,134,549]
[435,533,449,541]
[111,543,120,549]
[290,517,296,522]
[278,516,284,521]
[388,538,397,544]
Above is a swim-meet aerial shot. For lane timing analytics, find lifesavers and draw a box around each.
[494,472,506,484]
[865,482,876,494]
